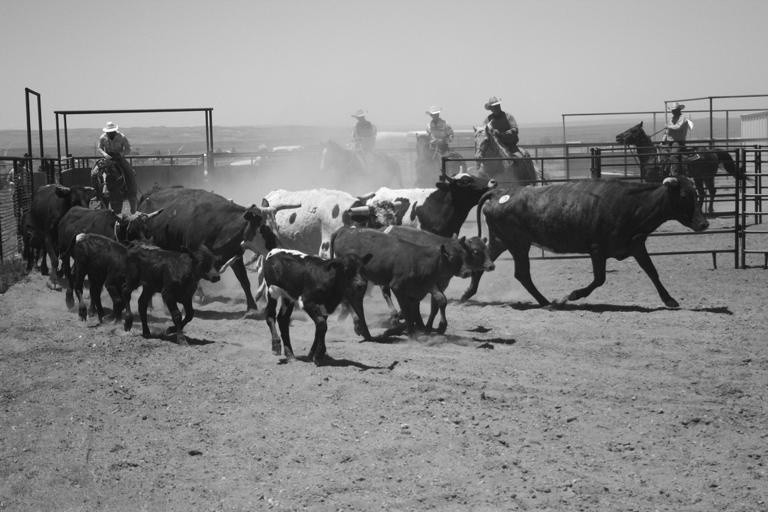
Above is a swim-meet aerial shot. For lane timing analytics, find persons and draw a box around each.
[348,107,376,153]
[6,159,33,219]
[94,121,132,160]
[423,105,454,152]
[659,103,692,179]
[480,95,519,155]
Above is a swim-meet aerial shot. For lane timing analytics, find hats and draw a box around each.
[102,122,119,134]
[424,105,444,116]
[667,102,686,112]
[350,109,367,119]
[484,96,504,110]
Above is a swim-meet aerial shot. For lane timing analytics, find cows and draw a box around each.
[458,174,710,308]
[18,174,498,366]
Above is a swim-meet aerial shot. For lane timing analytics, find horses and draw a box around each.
[472,125,538,186]
[415,132,468,187]
[316,138,402,187]
[94,161,138,214]
[615,120,752,213]
